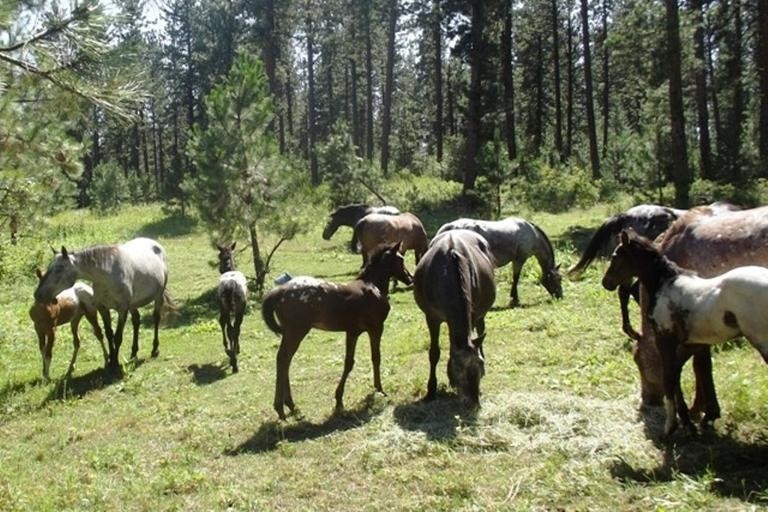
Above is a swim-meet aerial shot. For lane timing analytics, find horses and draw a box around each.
[322,203,401,269]
[260,239,414,420]
[601,226,768,441]
[412,228,499,408]
[350,211,429,292]
[28,267,110,378]
[434,215,564,309]
[33,237,185,380]
[215,241,248,373]
[633,205,768,422]
[566,199,742,343]
